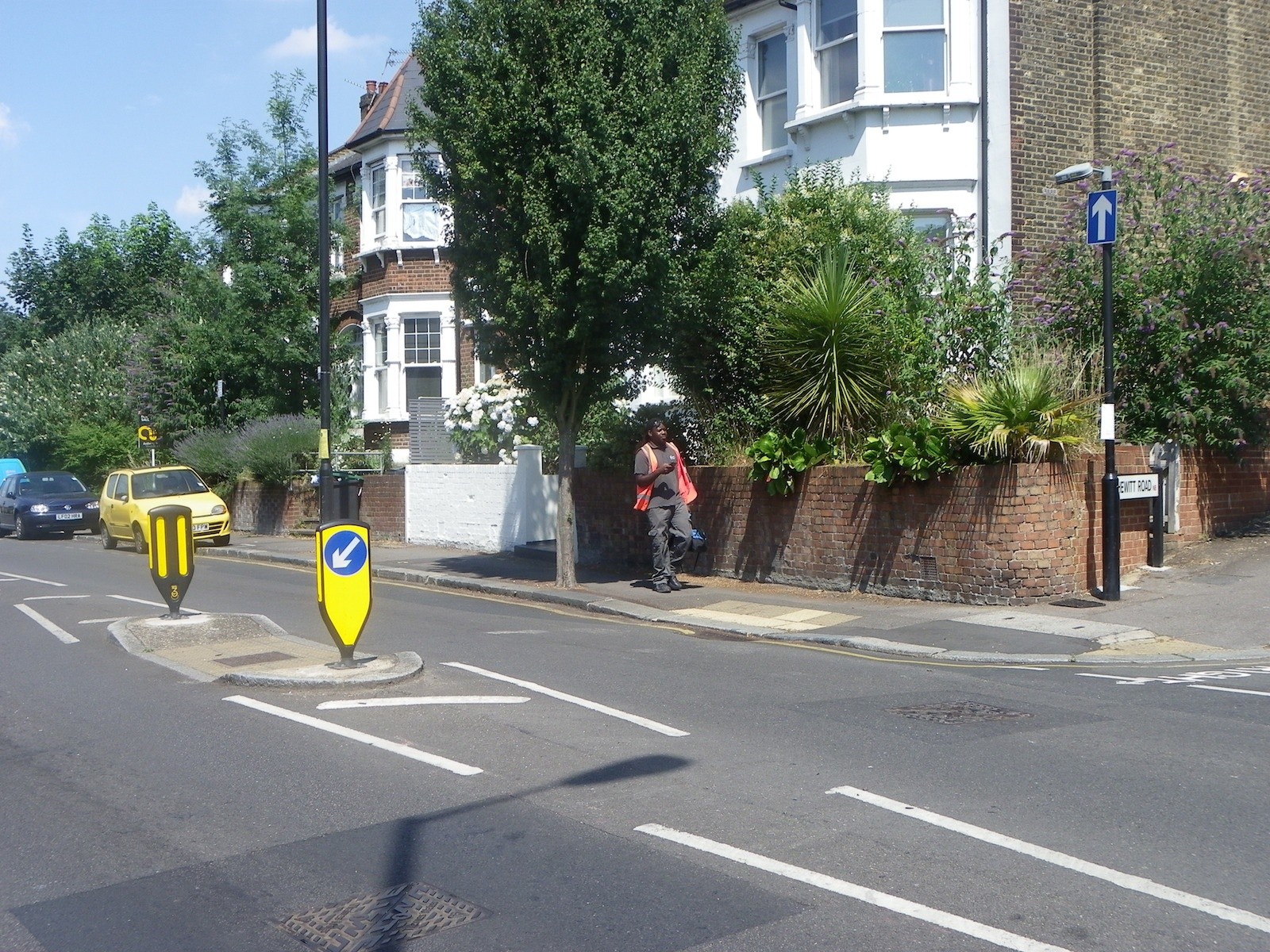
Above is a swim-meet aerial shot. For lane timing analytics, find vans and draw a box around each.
[0,458,32,494]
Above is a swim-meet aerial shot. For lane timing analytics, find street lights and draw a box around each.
[1054,162,1123,603]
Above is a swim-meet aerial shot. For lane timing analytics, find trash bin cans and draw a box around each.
[310,470,364,524]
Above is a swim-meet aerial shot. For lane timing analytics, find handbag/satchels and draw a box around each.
[688,520,708,552]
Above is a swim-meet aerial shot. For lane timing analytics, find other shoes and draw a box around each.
[667,577,682,590]
[652,583,671,592]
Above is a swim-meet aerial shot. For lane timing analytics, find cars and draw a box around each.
[97,465,232,555]
[0,470,102,541]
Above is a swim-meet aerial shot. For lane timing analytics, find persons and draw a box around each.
[633,417,698,593]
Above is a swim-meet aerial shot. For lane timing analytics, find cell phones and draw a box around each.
[669,462,678,466]
